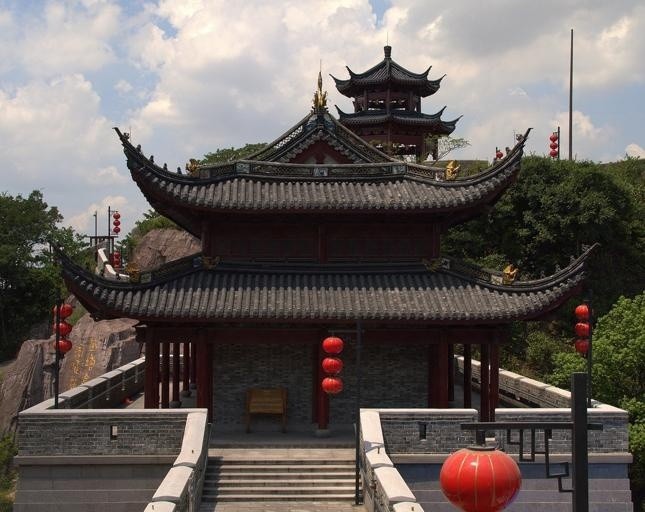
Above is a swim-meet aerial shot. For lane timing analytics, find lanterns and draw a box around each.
[575,304,590,353]
[496,152,503,158]
[438,446,522,512]
[53,304,72,353]
[322,336,344,395]
[114,251,120,268]
[113,213,120,233]
[550,134,558,157]
